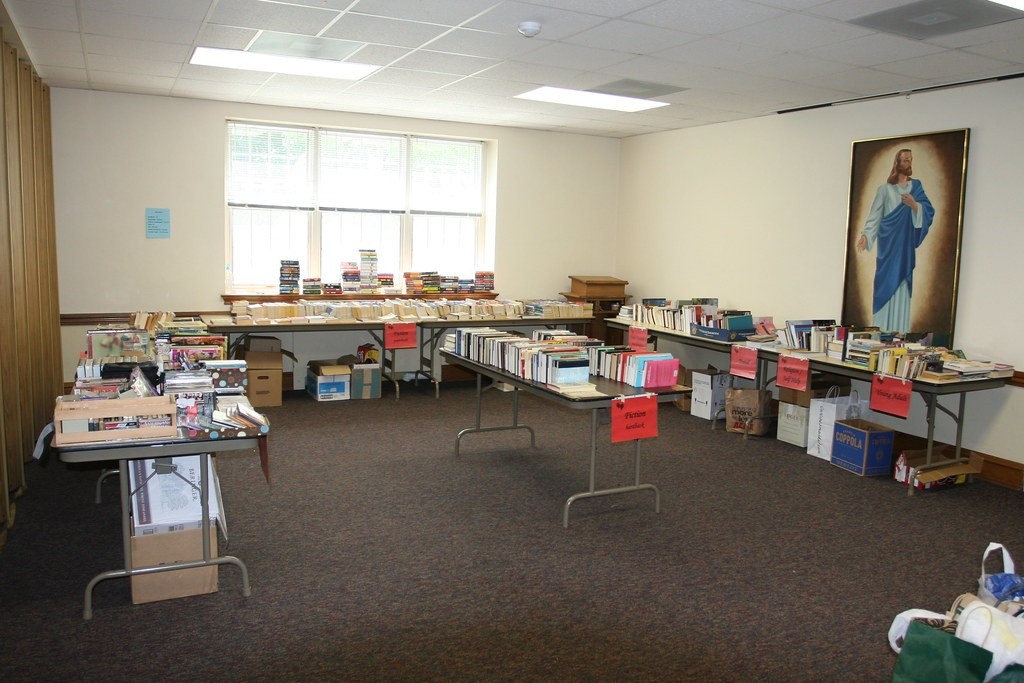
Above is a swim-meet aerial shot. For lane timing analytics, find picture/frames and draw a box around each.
[840,128,970,350]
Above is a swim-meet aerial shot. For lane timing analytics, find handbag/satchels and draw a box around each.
[806,385,872,462]
[885,542,1024,683]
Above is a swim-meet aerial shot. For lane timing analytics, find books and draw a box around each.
[211,296,594,324]
[279,248,494,294]
[616,298,1015,384]
[76,312,268,427]
[444,328,680,393]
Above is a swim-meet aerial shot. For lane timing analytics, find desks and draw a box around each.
[59,380,266,620]
[200,315,416,402]
[415,315,596,400]
[733,342,1014,497]
[438,347,693,531]
[603,316,745,431]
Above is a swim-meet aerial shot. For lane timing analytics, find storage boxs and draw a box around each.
[307,360,352,376]
[671,363,717,412]
[724,388,772,435]
[776,400,808,448]
[690,372,732,421]
[775,373,852,406]
[338,353,382,400]
[357,344,379,363]
[304,371,351,402]
[235,346,298,408]
[805,397,872,461]
[830,419,894,477]
[126,456,219,535]
[244,336,282,352]
[122,526,219,605]
[892,444,984,491]
